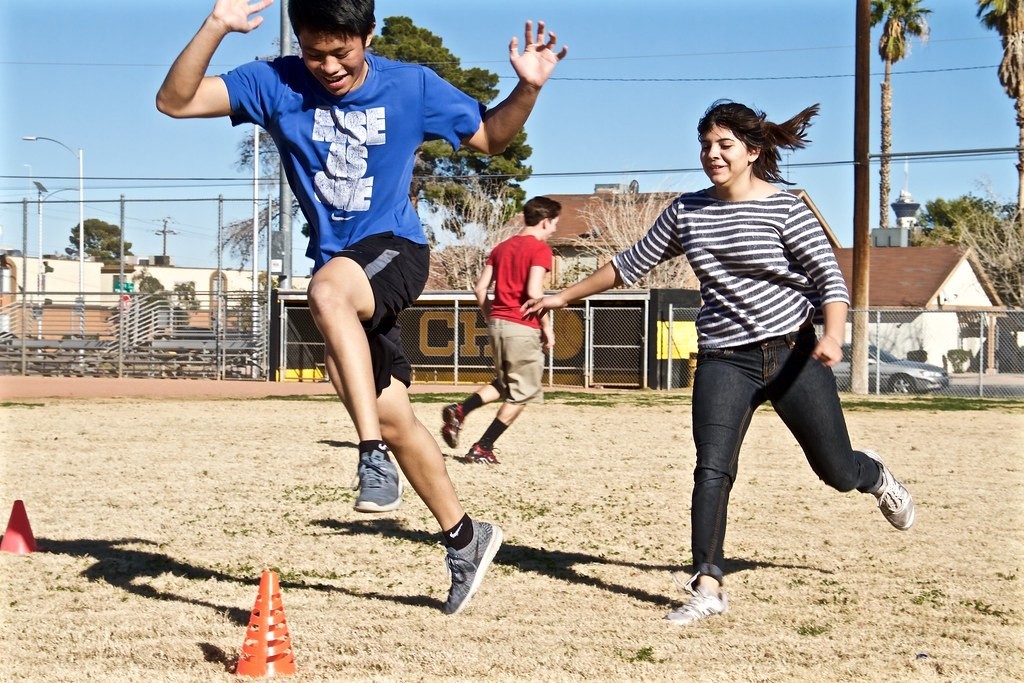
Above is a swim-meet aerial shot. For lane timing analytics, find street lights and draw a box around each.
[33,181,80,369]
[22,136,86,378]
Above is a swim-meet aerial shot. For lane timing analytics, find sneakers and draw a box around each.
[443,404,464,449]
[445,519,503,617]
[465,444,502,465]
[350,450,404,512]
[862,450,914,531]
[662,571,725,625]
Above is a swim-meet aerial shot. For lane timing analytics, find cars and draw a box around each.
[831,342,950,395]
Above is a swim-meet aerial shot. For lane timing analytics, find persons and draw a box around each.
[520,102,914,621]
[439,196,562,465]
[155,0,569,615]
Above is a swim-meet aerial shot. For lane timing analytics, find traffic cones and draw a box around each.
[0,499,39,555]
[233,570,297,678]
